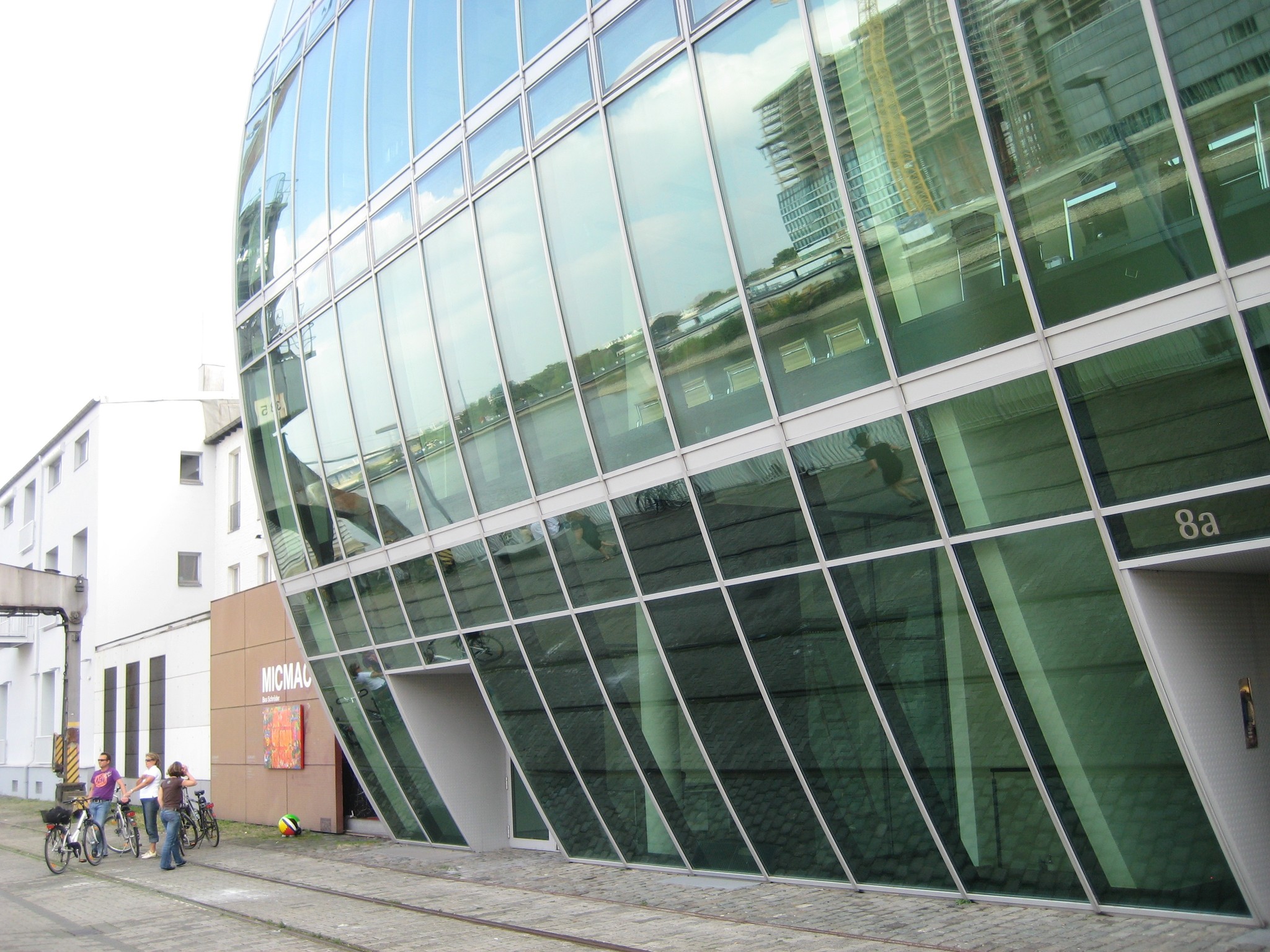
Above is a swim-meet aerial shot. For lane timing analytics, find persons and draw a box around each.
[126,753,161,860]
[850,433,925,506]
[349,654,396,704]
[84,752,128,859]
[157,761,197,870]
[564,511,623,563]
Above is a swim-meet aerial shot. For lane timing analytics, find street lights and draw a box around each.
[1061,67,1196,280]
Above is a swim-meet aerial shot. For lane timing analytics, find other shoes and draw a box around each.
[141,850,157,859]
[602,557,611,563]
[162,865,175,870]
[612,544,618,554]
[91,850,97,857]
[176,859,187,866]
[909,500,921,507]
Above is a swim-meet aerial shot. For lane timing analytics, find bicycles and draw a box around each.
[633,478,701,517]
[330,688,390,749]
[178,786,220,856]
[40,796,105,874]
[102,784,142,858]
[422,630,505,665]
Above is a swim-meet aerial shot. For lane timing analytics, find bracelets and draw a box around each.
[130,789,134,793]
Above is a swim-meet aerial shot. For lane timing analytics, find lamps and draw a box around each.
[75,574,85,592]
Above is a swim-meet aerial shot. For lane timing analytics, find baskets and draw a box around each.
[40,810,72,823]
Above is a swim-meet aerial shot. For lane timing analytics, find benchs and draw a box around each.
[479,528,576,565]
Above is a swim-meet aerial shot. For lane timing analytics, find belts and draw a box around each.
[163,808,180,812]
[93,799,110,803]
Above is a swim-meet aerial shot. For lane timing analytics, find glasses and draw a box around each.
[98,759,108,762]
[356,665,360,670]
[146,759,153,761]
